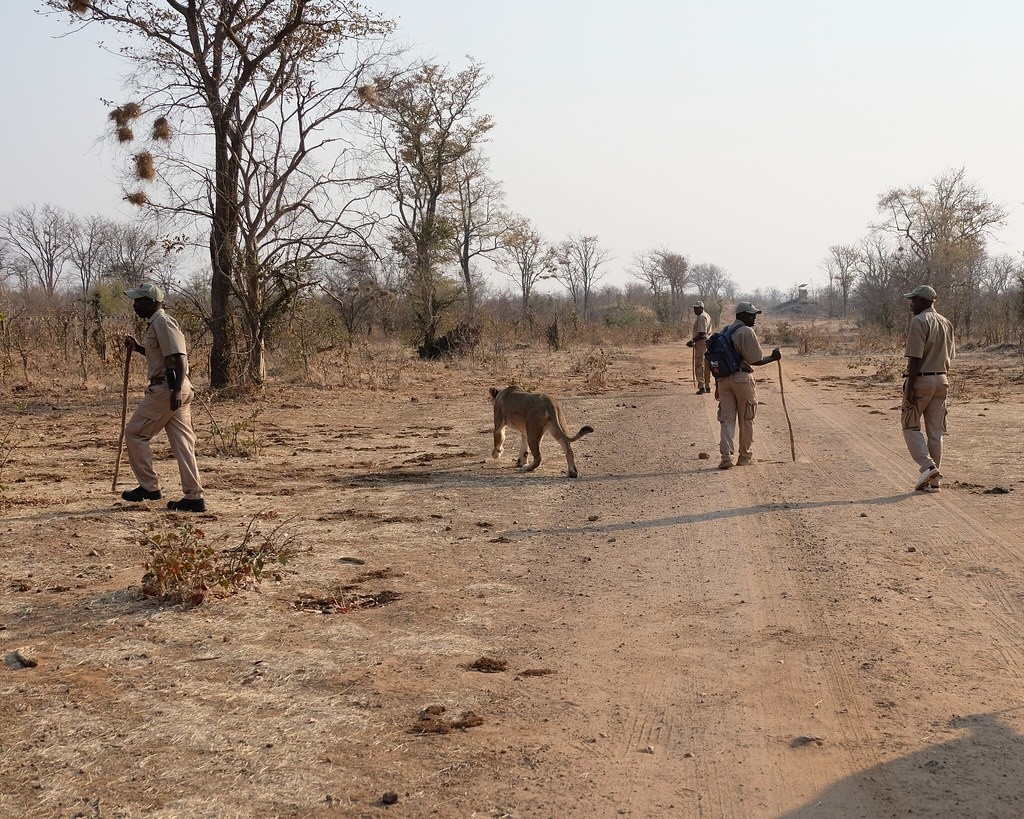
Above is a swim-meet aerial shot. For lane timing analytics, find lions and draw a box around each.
[488,385,594,478]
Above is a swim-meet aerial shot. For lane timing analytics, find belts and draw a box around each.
[902,372,945,378]
[733,368,750,373]
[147,377,167,387]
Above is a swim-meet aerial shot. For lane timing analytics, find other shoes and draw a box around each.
[697,387,705,395]
[122,486,162,502]
[718,461,734,468]
[737,455,757,465]
[929,485,941,493]
[167,497,205,511]
[704,388,711,392]
[915,466,938,490]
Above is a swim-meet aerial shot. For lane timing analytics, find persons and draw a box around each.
[714,302,784,468]
[686,300,714,395]
[900,287,957,493]
[120,282,209,513]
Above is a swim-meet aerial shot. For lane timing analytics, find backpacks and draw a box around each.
[705,323,747,378]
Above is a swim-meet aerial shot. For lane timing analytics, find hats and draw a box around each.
[903,285,937,302]
[692,301,704,309]
[124,283,164,303]
[735,302,762,314]
